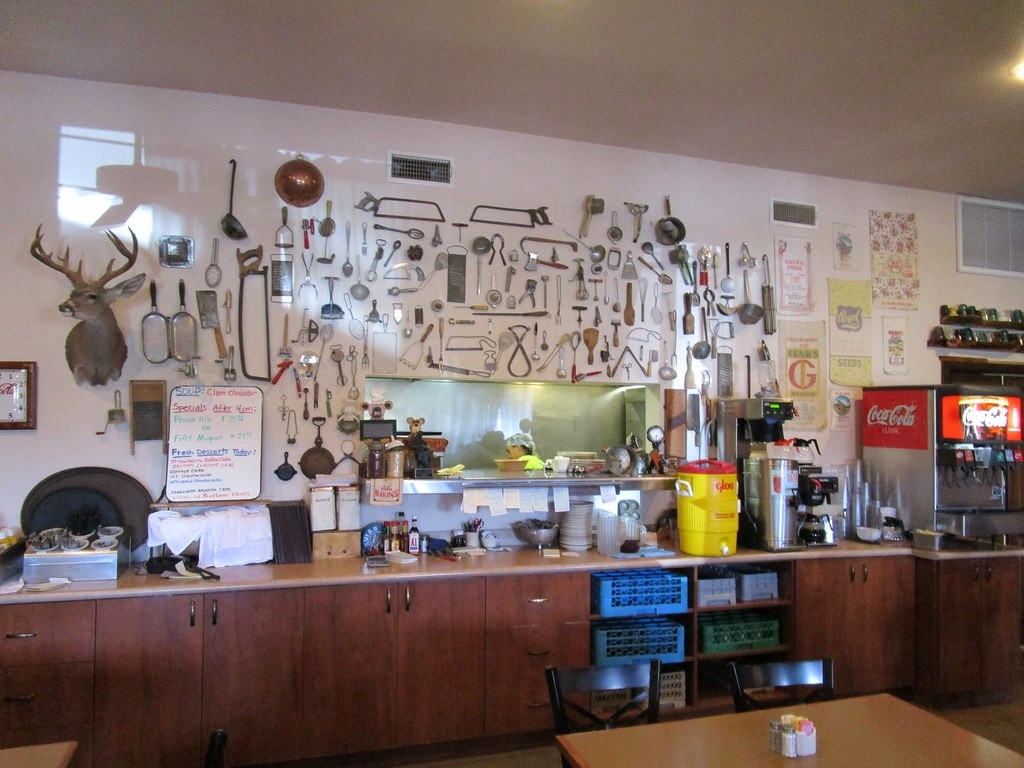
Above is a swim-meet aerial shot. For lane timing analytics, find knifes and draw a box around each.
[196,291,226,358]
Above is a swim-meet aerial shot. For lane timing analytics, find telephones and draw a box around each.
[146,555,192,574]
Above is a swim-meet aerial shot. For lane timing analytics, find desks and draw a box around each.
[554,692,1024,768]
[0,739,78,768]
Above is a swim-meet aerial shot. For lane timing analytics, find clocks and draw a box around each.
[0,361,37,430]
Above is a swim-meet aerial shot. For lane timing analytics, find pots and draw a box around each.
[274,452,297,481]
[300,417,335,478]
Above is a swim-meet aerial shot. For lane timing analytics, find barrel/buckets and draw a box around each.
[743,458,800,552]
[675,460,741,557]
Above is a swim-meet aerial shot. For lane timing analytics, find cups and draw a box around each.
[823,458,881,541]
[30,526,124,552]
[931,303,1024,343]
[465,532,479,548]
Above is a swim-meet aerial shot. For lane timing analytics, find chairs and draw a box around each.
[728,657,833,713]
[543,657,662,768]
[204,728,228,768]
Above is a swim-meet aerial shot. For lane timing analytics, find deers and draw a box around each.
[29,223,146,387]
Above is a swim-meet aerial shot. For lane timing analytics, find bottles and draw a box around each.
[385,435,405,478]
[769,720,781,753]
[366,441,385,479]
[383,512,420,555]
[780,724,798,759]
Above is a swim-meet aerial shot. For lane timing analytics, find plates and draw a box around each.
[559,499,594,552]
[558,452,605,468]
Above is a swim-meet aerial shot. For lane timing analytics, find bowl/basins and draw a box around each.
[481,538,497,547]
[494,459,529,471]
[856,527,882,541]
[519,526,559,548]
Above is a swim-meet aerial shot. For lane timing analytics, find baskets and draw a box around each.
[592,571,779,713]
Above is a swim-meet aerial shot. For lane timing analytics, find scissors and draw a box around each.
[474,518,485,531]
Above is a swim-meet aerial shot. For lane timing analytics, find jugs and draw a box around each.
[767,441,795,461]
[882,517,905,541]
[789,438,822,465]
[598,511,647,555]
[796,512,834,544]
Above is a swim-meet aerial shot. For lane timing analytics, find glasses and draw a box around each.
[507,446,516,454]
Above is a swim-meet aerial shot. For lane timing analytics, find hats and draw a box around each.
[506,433,534,454]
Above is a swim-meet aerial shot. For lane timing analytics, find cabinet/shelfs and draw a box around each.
[792,554,916,701]
[0,599,96,768]
[93,587,303,768]
[303,576,484,764]
[915,556,1024,711]
[484,570,590,738]
[588,560,798,725]
[925,316,1024,361]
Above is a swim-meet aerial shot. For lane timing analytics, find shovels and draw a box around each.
[684,293,694,334]
[685,346,696,389]
[583,328,599,365]
[624,283,635,326]
[374,224,449,289]
[490,333,515,374]
[638,257,673,285]
[344,293,364,340]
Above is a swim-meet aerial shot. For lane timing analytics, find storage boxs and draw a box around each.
[590,618,685,668]
[570,458,608,473]
[556,450,597,459]
[636,670,686,714]
[910,530,945,552]
[590,569,689,617]
[795,727,817,757]
[22,527,132,581]
[697,577,737,608]
[310,486,338,532]
[699,617,780,656]
[336,484,361,532]
[591,689,632,715]
[310,529,362,561]
[642,522,671,545]
[701,564,778,602]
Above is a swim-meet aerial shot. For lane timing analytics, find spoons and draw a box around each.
[341,220,735,379]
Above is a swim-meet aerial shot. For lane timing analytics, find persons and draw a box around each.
[506,433,545,470]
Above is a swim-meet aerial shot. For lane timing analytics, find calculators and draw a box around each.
[366,555,390,567]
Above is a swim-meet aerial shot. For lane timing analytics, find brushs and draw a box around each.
[581,198,604,237]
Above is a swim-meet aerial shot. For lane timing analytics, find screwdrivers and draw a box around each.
[452,306,489,309]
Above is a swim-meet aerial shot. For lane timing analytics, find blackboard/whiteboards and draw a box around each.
[165,386,263,502]
